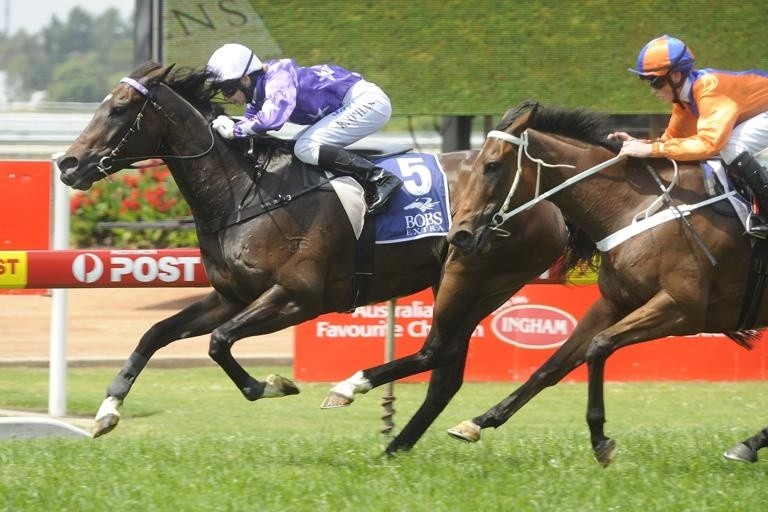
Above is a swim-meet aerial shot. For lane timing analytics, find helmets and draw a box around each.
[628,35,695,77]
[205,44,264,84]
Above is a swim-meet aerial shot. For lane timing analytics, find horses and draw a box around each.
[57,59,569,464]
[446,100,768,467]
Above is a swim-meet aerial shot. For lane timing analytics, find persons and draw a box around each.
[204,42,405,217]
[605,36,768,236]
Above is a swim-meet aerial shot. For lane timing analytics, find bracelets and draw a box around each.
[652,141,660,158]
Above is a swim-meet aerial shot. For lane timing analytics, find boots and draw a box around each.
[319,145,404,214]
[725,151,768,233]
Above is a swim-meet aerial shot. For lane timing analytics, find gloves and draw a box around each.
[211,114,236,141]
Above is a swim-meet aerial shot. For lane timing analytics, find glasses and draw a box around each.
[222,82,236,98]
[652,76,665,91]
